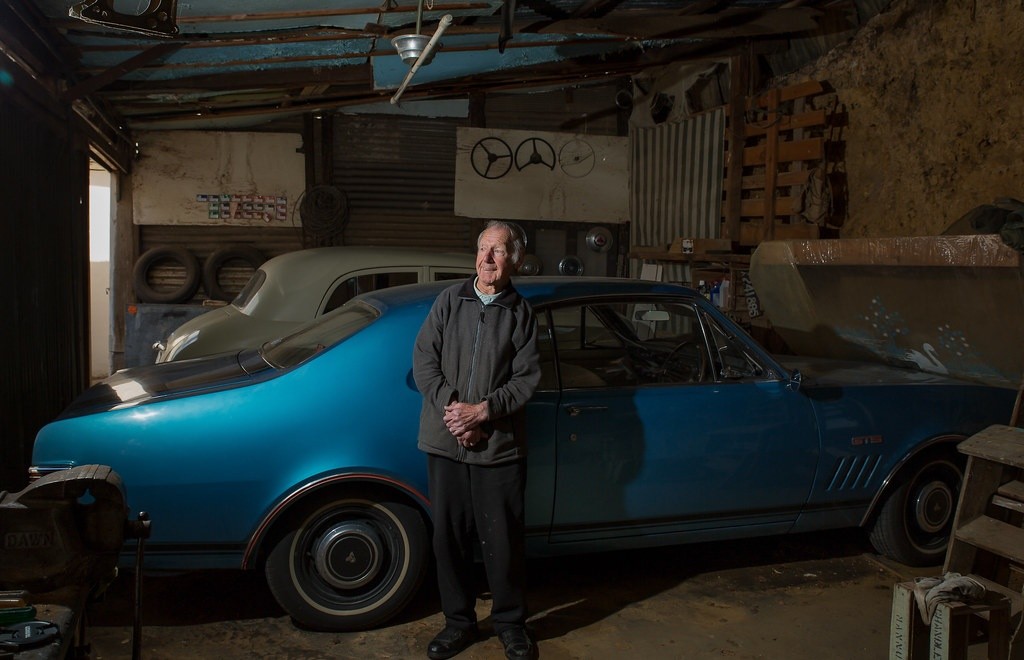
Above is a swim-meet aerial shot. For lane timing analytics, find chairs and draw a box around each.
[537,326,607,391]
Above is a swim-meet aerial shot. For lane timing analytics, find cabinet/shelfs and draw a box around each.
[941,422,1024,660]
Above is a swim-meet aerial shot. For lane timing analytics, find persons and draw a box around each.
[412,221,547,660]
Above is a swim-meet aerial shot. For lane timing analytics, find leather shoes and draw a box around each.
[498,627,540,660]
[427,626,479,660]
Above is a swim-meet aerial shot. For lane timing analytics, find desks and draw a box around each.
[14,569,87,660]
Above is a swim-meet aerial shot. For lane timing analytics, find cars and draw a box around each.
[26,277,1024,633]
[151,246,477,368]
